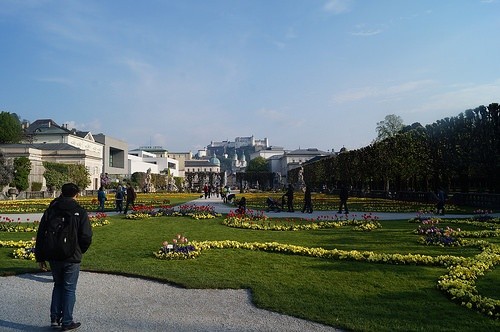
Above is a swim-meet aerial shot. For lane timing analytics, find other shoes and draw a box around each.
[63,322,81,332]
[51,323,62,330]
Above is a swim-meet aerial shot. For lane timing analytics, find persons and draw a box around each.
[228,194,236,202]
[237,197,246,211]
[337,183,349,214]
[300,185,314,214]
[283,184,295,212]
[123,183,137,213]
[97,185,107,213]
[436,186,445,216]
[204,182,231,204]
[143,184,148,194]
[113,184,127,214]
[35,183,92,332]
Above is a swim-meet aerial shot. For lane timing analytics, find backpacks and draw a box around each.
[37,207,80,259]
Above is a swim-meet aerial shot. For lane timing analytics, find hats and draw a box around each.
[62,183,79,197]
